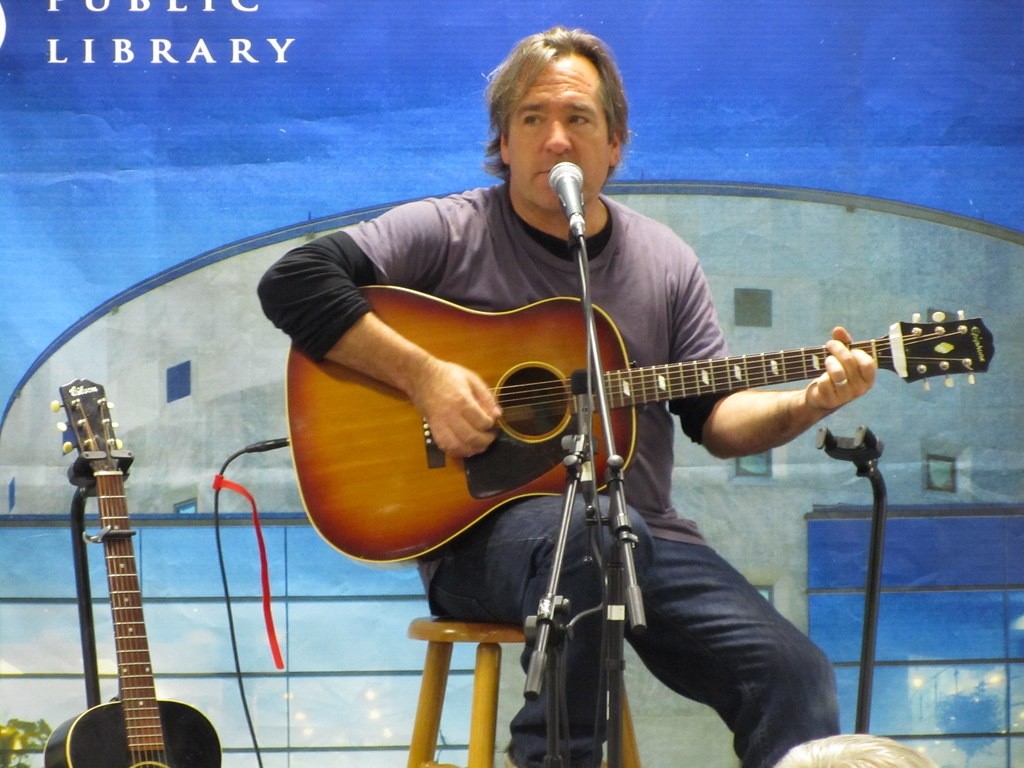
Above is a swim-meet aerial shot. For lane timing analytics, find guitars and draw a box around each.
[41,376,223,768]
[281,282,996,568]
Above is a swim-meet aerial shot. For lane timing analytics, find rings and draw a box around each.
[835,379,848,386]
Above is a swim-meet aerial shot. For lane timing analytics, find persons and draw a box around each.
[257,21,875,768]
[772,733,937,768]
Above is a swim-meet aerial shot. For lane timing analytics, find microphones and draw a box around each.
[547,161,586,238]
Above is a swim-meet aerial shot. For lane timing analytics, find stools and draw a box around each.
[406,617,643,768]
